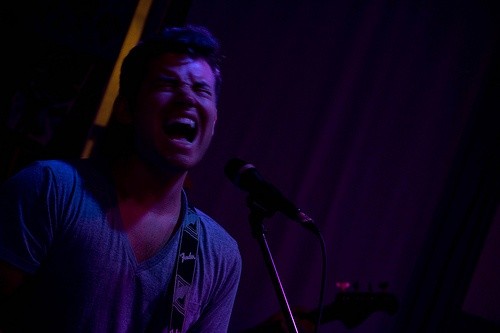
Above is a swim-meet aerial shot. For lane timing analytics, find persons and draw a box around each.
[0,24,244,333]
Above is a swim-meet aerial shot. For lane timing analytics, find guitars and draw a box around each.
[240,281,400,333]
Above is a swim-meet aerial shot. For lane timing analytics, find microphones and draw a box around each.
[225,157,326,236]
